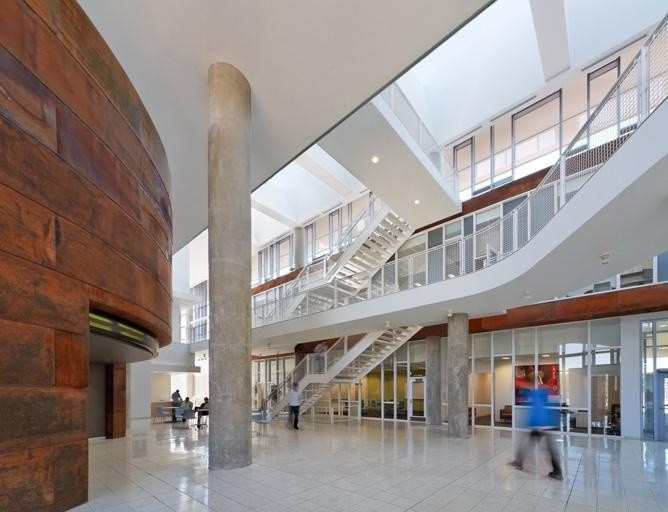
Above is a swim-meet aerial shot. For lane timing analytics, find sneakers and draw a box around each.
[546,470,562,481]
[293,426,299,430]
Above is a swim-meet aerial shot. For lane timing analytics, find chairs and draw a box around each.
[154,406,188,425]
[256,408,274,440]
[499,405,512,422]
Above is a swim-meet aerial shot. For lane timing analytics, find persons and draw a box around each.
[171,389,183,423]
[180,396,193,422]
[312,339,329,374]
[196,397,209,428]
[287,382,303,431]
[508,372,564,481]
[269,384,281,418]
